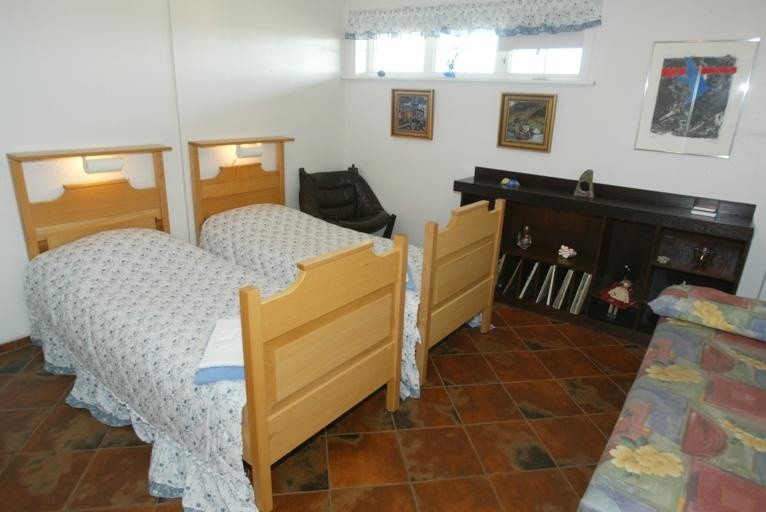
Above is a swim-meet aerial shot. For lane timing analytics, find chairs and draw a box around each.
[299,164,397,239]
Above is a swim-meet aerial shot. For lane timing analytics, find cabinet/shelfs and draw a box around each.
[454,166,756,345]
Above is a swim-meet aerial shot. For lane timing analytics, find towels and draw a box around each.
[405,264,413,291]
[196,317,244,384]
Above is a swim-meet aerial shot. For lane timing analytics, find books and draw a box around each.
[494,253,594,317]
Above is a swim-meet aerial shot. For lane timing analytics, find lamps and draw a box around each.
[82,156,124,172]
[236,145,263,157]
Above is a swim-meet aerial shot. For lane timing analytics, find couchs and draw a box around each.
[577,285,766,512]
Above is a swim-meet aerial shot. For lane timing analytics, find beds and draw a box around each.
[187,136,506,403]
[7,144,408,512]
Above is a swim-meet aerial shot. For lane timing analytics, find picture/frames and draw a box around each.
[634,40,760,158]
[498,93,557,152]
[391,89,434,140]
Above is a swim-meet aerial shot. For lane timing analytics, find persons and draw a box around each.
[597,264,639,324]
